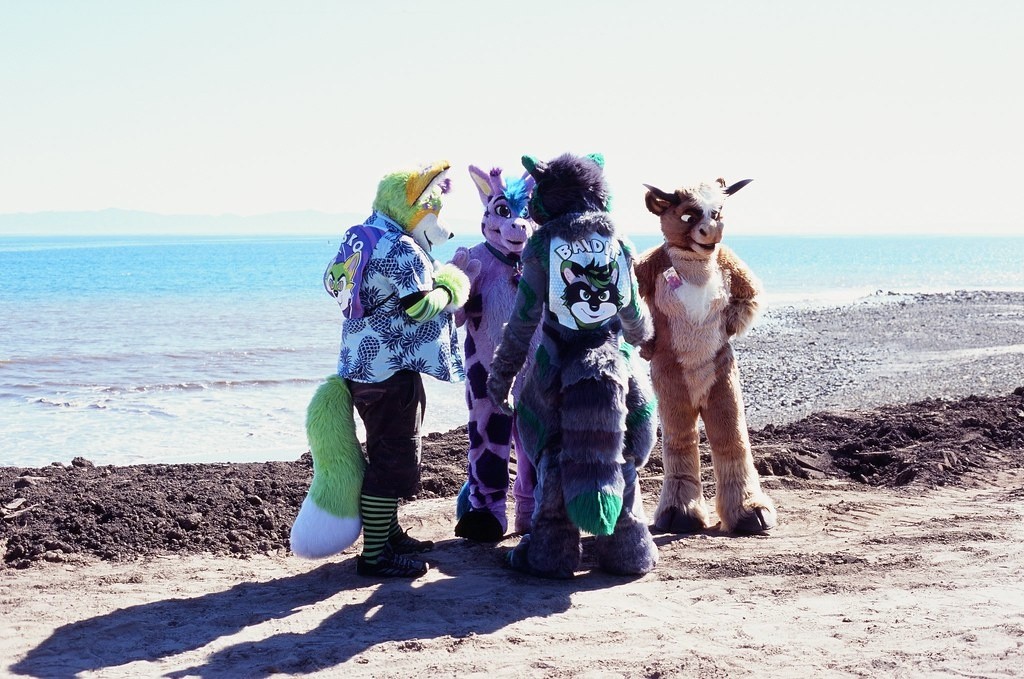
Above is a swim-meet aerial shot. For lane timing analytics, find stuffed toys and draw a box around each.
[289,153,656,579]
[632,177,781,533]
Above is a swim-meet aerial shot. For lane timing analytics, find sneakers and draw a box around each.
[357,543,429,578]
[387,527,433,554]
